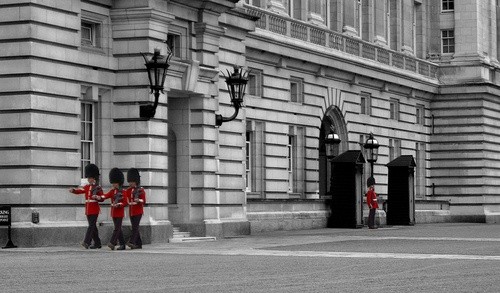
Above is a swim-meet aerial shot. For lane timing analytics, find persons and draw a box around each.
[366,177,379,229]
[68,164,105,249]
[96,167,127,250]
[126,168,146,249]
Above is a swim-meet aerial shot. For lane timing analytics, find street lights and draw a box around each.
[364,132,380,176]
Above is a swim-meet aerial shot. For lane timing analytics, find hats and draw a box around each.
[367,177,375,187]
[109,168,124,186]
[85,164,99,181]
[127,168,140,185]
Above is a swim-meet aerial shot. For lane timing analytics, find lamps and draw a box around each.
[139,48,173,118]
[325,123,342,144]
[216,66,253,126]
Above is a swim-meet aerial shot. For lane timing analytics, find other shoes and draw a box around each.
[369,226,378,229]
[82,242,89,249]
[108,243,115,250]
[117,244,125,250]
[90,244,102,249]
[128,243,142,249]
